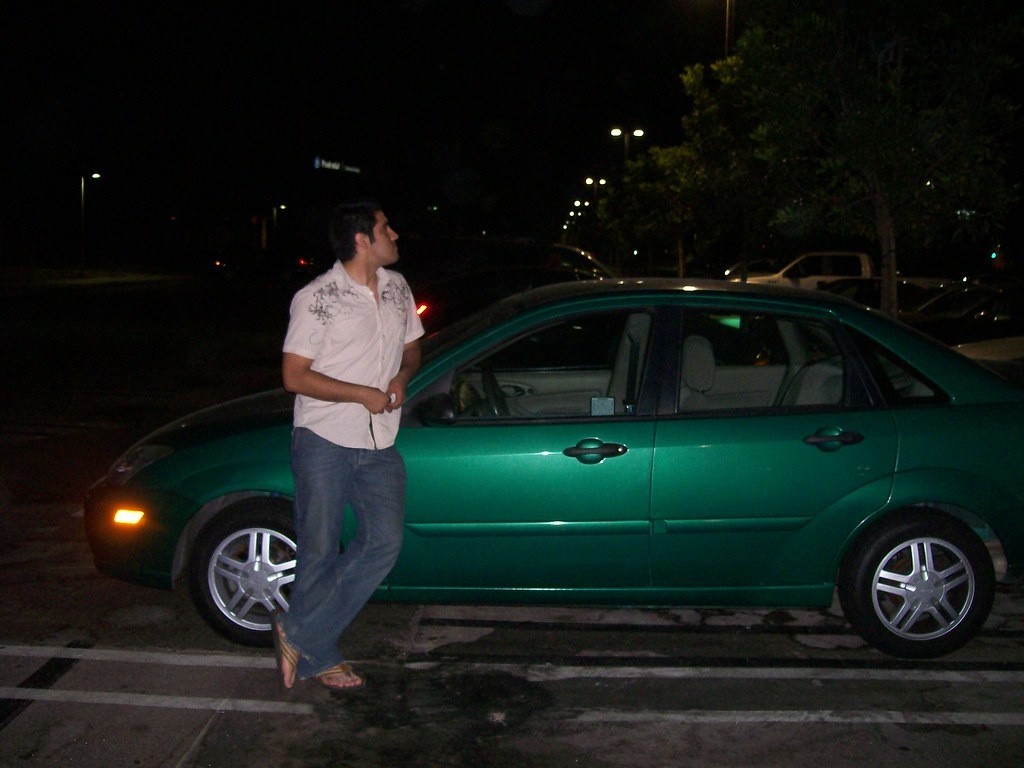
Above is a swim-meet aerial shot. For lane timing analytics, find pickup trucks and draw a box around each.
[729,249,940,295]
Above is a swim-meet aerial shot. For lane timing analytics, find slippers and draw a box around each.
[309,661,368,693]
[270,608,299,687]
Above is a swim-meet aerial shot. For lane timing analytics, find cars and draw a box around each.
[85,277,1024,660]
[269,234,616,336]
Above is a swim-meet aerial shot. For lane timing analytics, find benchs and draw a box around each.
[780,356,934,409]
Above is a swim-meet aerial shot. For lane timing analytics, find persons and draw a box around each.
[271,203,425,692]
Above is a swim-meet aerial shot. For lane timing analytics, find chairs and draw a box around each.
[607,311,652,415]
[680,335,714,410]
